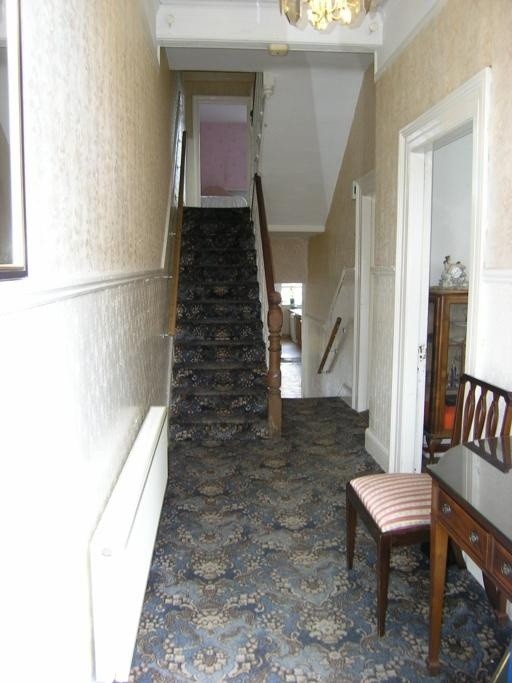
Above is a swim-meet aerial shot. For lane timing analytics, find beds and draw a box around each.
[200,186,247,208]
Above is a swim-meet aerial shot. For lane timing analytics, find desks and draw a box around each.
[425,435,512,675]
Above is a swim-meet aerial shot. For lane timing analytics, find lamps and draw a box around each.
[279,0,376,36]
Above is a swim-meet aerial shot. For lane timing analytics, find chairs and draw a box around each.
[345,374,512,638]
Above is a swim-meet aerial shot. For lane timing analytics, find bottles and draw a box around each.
[451,363,456,388]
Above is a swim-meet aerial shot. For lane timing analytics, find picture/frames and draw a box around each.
[0,1,27,279]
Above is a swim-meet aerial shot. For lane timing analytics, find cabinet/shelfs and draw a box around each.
[422,287,469,464]
[294,314,301,352]
